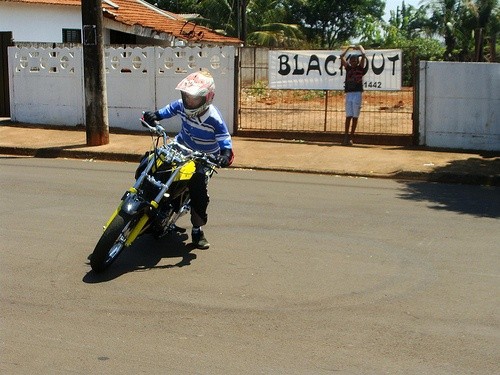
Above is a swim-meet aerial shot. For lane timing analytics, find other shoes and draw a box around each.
[191,230,210,248]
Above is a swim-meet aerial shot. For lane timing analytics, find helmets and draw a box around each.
[175,71,216,118]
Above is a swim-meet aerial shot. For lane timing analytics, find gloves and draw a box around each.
[139,110,162,128]
[212,149,234,168]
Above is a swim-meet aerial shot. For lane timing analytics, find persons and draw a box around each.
[339,45,369,146]
[136,71,235,250]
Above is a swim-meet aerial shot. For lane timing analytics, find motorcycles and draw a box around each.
[90,109,220,275]
